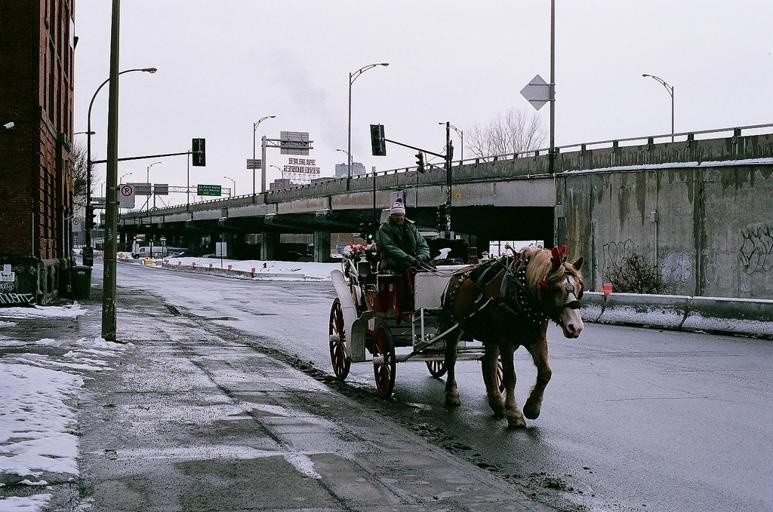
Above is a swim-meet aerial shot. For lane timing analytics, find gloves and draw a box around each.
[411,255,427,266]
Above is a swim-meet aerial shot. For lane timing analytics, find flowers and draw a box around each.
[342,242,376,258]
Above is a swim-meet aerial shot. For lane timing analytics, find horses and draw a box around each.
[439,246,585,429]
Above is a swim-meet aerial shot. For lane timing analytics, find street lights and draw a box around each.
[642,73,674,142]
[252,115,277,196]
[223,148,354,198]
[119,161,163,184]
[85,66,158,247]
[346,61,389,192]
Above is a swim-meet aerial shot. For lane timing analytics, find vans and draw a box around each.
[131,246,168,260]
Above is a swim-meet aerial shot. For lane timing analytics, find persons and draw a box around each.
[378,196,430,315]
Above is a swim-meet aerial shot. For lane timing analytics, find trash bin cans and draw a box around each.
[82,247,93,266]
[72,266,92,300]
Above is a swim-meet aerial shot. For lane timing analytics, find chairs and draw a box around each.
[358,261,400,293]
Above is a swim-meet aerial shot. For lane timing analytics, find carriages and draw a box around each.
[328,241,587,428]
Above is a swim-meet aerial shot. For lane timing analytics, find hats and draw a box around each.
[390,198,406,215]
